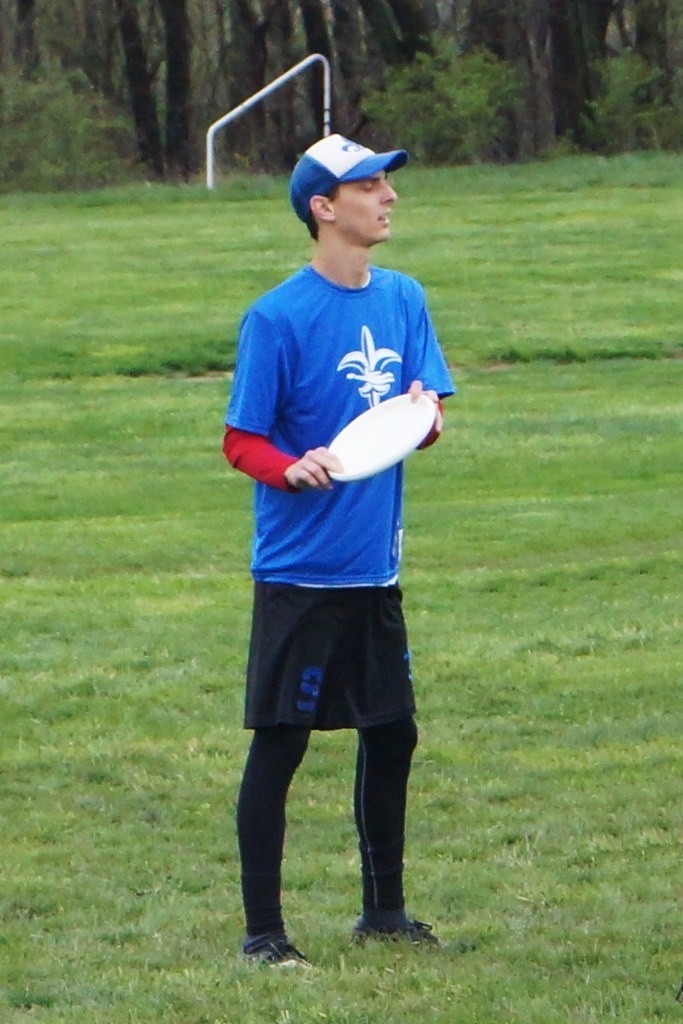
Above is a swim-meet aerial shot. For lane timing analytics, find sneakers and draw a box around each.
[238,935,323,974]
[349,917,445,948]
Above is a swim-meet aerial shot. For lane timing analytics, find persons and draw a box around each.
[221,134,455,970]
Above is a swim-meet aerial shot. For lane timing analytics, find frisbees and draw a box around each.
[326,392,436,483]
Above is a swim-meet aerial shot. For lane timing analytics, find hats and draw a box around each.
[289,134,409,221]
